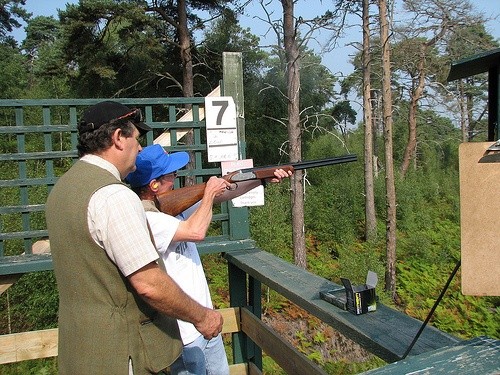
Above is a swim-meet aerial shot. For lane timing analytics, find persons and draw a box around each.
[45,101,223,375]
[128,144,293,375]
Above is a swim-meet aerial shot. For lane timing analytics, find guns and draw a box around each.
[154,154,358,217]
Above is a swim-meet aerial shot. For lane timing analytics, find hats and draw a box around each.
[79,100,153,136]
[125,144,189,187]
